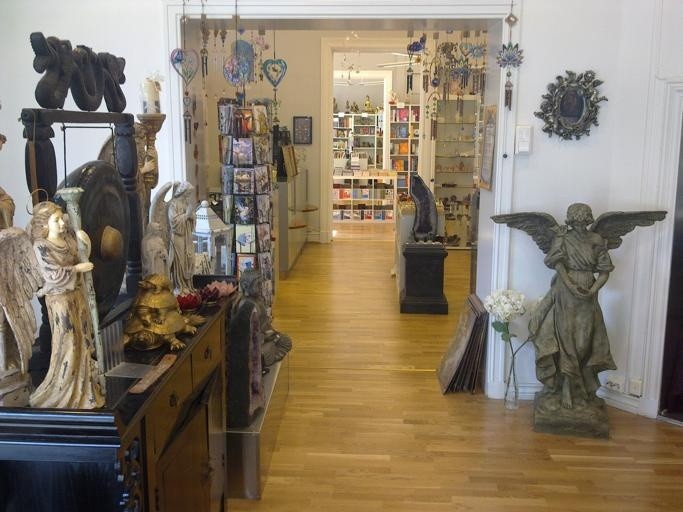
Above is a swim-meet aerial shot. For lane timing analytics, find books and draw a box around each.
[218,102,274,301]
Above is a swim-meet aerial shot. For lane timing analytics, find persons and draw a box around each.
[28,201,104,409]
[166,182,195,292]
[139,221,169,281]
[529,201,617,414]
[236,268,292,364]
[130,122,158,220]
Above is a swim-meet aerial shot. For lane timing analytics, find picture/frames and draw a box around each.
[292,115,312,144]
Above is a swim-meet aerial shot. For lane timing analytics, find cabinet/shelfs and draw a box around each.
[332,174,398,224]
[385,104,421,194]
[275,166,312,273]
[332,114,385,169]
[428,93,478,250]
[0,298,229,511]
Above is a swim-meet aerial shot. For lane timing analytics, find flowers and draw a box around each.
[482,284,544,406]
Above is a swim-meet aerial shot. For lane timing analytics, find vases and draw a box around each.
[503,353,521,410]
